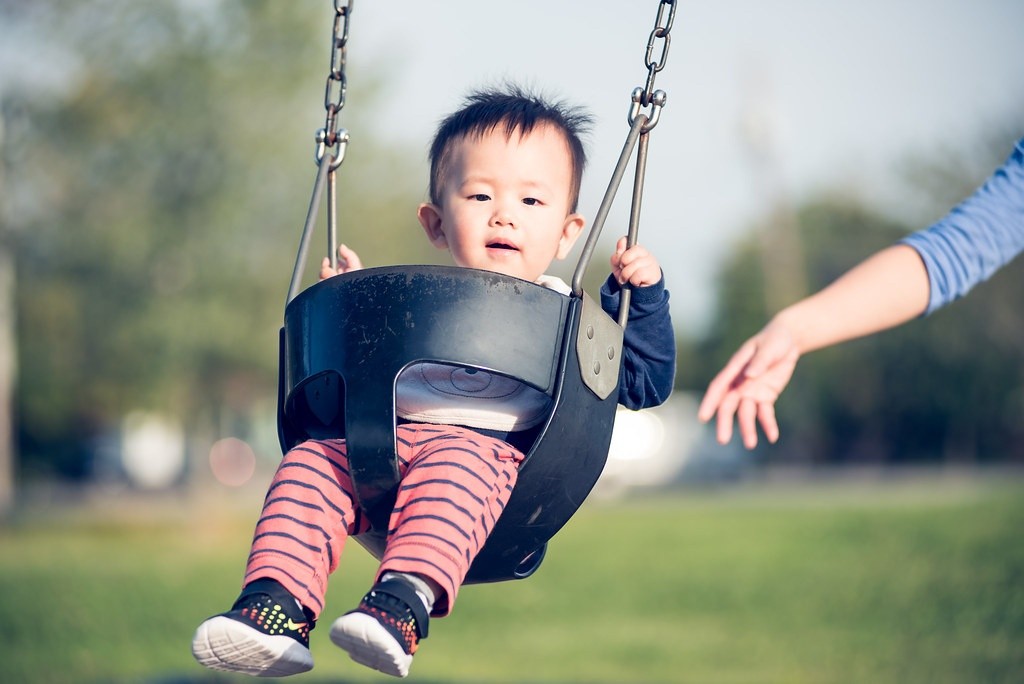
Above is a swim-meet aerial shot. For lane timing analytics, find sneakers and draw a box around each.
[191,580,313,677]
[327,579,431,678]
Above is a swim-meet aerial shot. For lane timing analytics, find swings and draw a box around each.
[276,1,677,587]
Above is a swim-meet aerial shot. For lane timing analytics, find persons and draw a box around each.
[697,128,1023,450]
[191,80,679,679]
